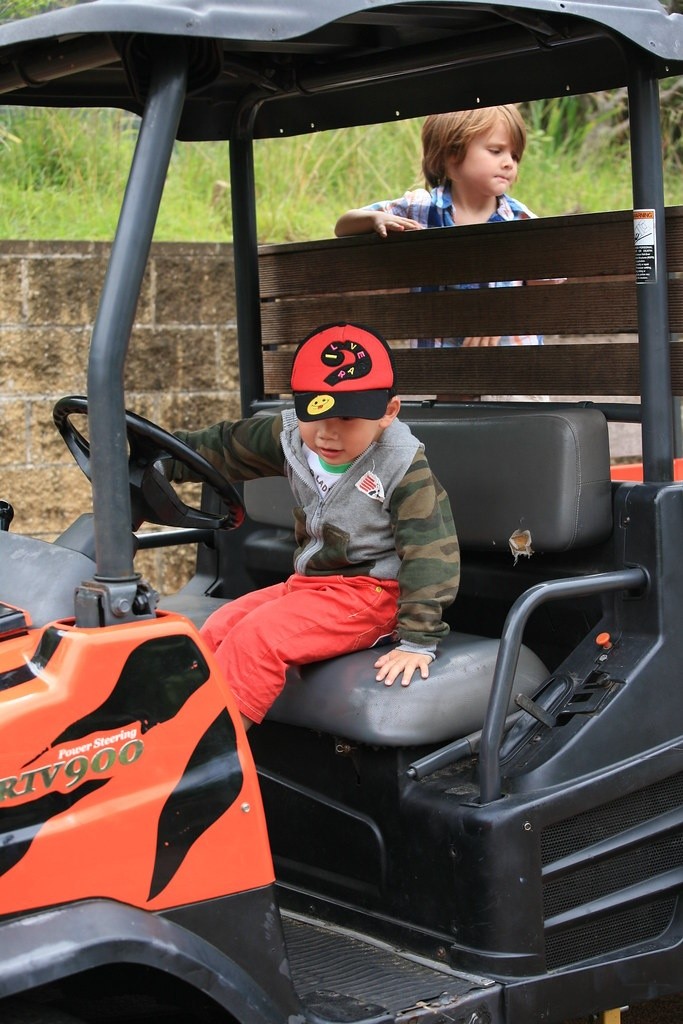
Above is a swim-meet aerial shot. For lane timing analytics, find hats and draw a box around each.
[291,322,398,422]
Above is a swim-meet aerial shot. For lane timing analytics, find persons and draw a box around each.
[156,321,459,735]
[334,104,549,402]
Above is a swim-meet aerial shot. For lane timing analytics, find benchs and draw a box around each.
[153,409,610,748]
